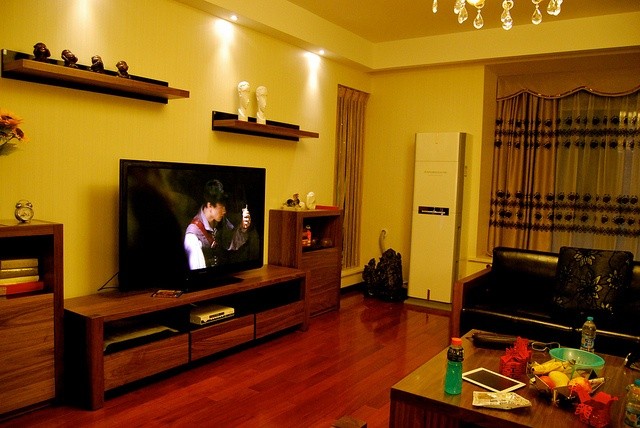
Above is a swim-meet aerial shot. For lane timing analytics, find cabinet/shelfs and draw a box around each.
[0,47,190,104]
[0,222,65,420]
[269,205,344,318]
[212,110,319,141]
[62,267,311,410]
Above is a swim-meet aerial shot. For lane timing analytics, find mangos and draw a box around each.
[549,371,569,387]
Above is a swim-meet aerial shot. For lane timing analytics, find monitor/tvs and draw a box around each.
[117,155,267,294]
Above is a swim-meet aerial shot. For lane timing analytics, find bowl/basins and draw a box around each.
[549,347,606,369]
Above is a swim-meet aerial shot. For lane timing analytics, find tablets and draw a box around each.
[461,365,526,394]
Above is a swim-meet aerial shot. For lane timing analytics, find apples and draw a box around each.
[569,377,592,393]
[540,375,555,389]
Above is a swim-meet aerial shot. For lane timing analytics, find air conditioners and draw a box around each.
[407,132,466,304]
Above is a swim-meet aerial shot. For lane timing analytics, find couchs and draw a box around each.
[450,247,640,357]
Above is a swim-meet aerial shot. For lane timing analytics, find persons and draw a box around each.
[182,176,254,269]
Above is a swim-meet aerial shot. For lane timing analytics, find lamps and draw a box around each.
[432,0,563,31]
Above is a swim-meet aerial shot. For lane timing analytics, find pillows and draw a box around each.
[553,246,633,319]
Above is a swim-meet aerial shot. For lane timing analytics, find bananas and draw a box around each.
[529,358,575,375]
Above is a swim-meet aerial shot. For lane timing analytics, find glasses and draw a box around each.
[529,341,561,353]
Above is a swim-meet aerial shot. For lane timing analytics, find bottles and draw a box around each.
[443,337,465,396]
[579,316,596,353]
[623,379,640,428]
